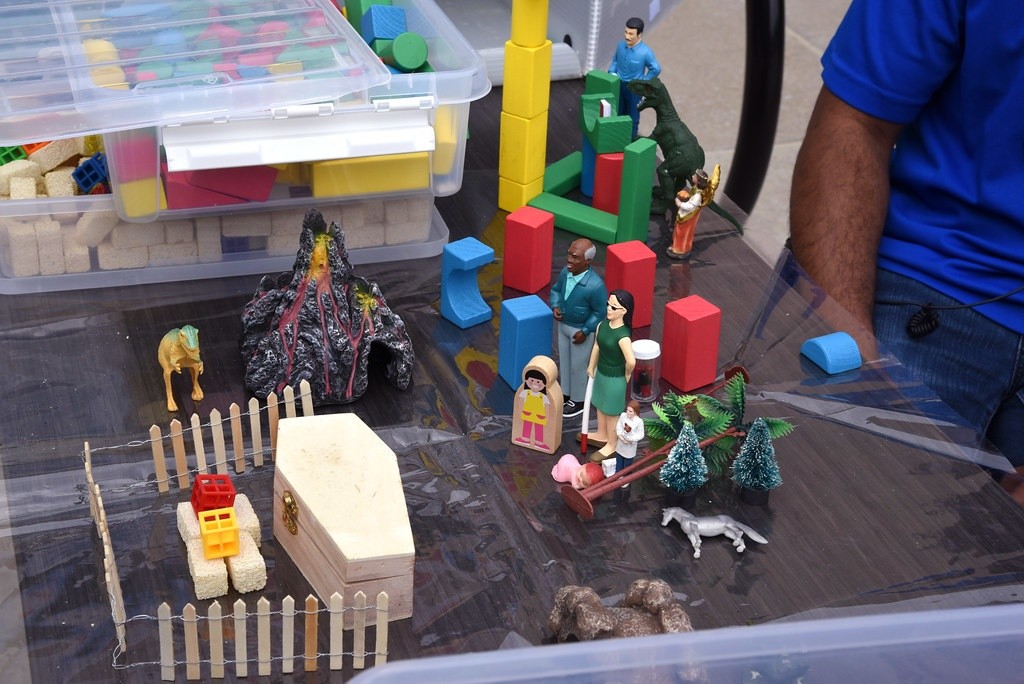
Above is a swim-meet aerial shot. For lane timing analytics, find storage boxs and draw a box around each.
[0,0,495,299]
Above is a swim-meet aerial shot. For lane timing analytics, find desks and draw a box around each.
[0,73,1024,683]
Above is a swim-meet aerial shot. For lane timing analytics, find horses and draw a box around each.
[661,506,769,559]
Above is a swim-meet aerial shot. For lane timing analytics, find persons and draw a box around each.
[670,168,708,255]
[614,399,645,488]
[608,17,660,142]
[551,453,604,490]
[576,289,635,463]
[549,238,609,417]
[789,0,1024,508]
